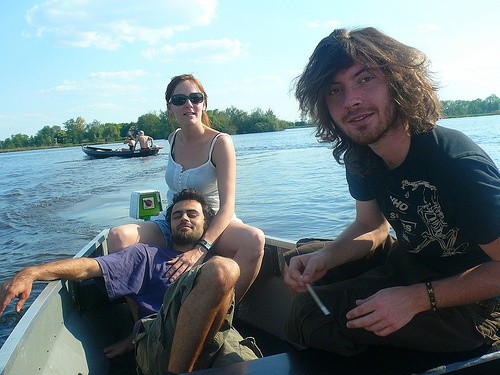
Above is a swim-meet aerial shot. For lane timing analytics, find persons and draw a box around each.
[103,74,266,359]
[0,187,263,375]
[282,27,500,357]
[124,131,153,151]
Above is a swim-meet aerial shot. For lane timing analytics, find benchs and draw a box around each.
[173,352,423,375]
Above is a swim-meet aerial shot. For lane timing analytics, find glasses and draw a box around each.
[168,93,203,107]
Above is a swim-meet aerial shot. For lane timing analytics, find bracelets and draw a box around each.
[426,281,437,311]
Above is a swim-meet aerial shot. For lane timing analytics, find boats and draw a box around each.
[82,145,164,159]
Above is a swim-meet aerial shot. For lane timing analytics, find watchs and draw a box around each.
[197,239,213,253]
